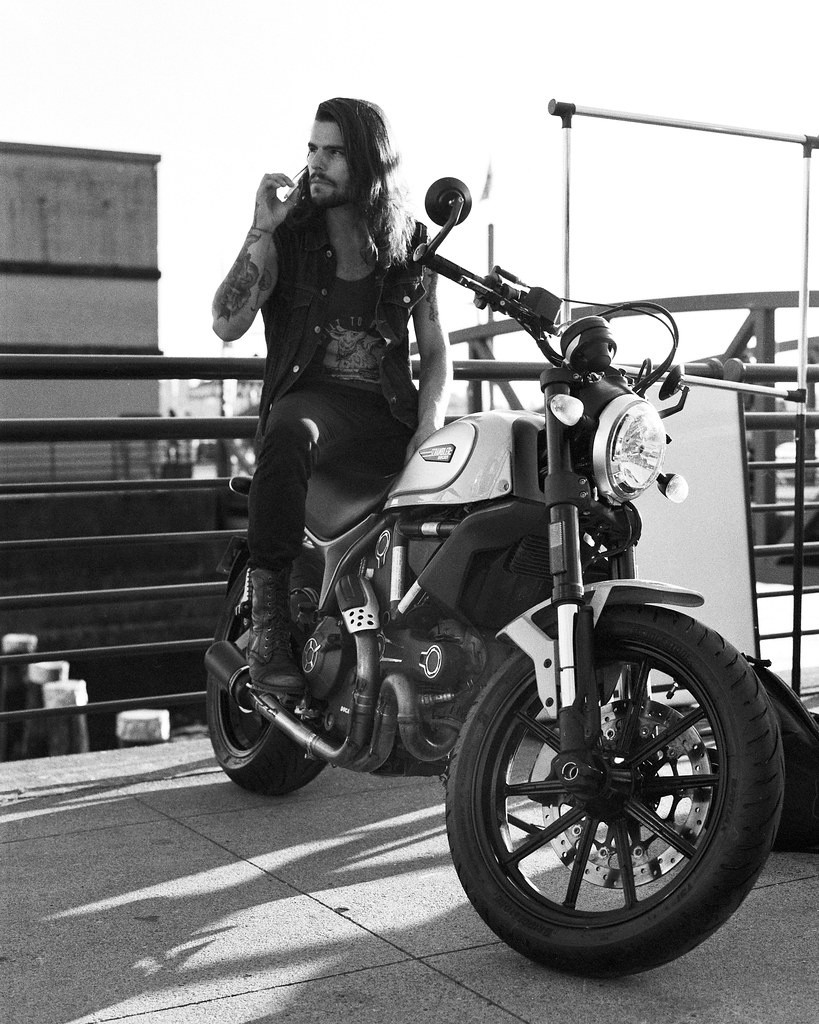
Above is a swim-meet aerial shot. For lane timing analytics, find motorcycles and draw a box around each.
[201,174,785,981]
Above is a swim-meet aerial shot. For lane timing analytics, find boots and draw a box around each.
[247,566,306,691]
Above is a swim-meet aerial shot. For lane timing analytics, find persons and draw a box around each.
[213,97,454,695]
